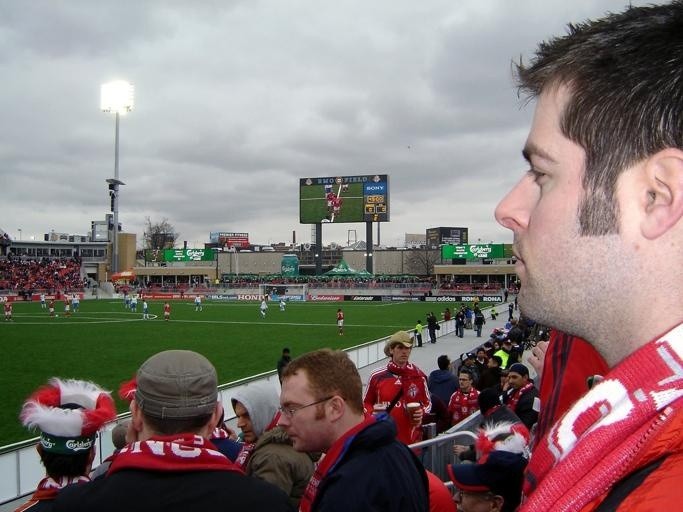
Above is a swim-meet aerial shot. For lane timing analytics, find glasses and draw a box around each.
[277,395,345,419]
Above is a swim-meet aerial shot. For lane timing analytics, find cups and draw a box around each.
[406,402,420,425]
[373,404,387,412]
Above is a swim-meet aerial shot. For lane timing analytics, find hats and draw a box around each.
[383,331,413,357]
[447,451,527,493]
[461,353,474,362]
[508,363,528,378]
[134,349,217,420]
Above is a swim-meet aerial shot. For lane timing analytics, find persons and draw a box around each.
[0,2,683,512]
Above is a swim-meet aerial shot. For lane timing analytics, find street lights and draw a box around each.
[99,73,137,276]
[31,235,36,240]
[18,228,22,239]
